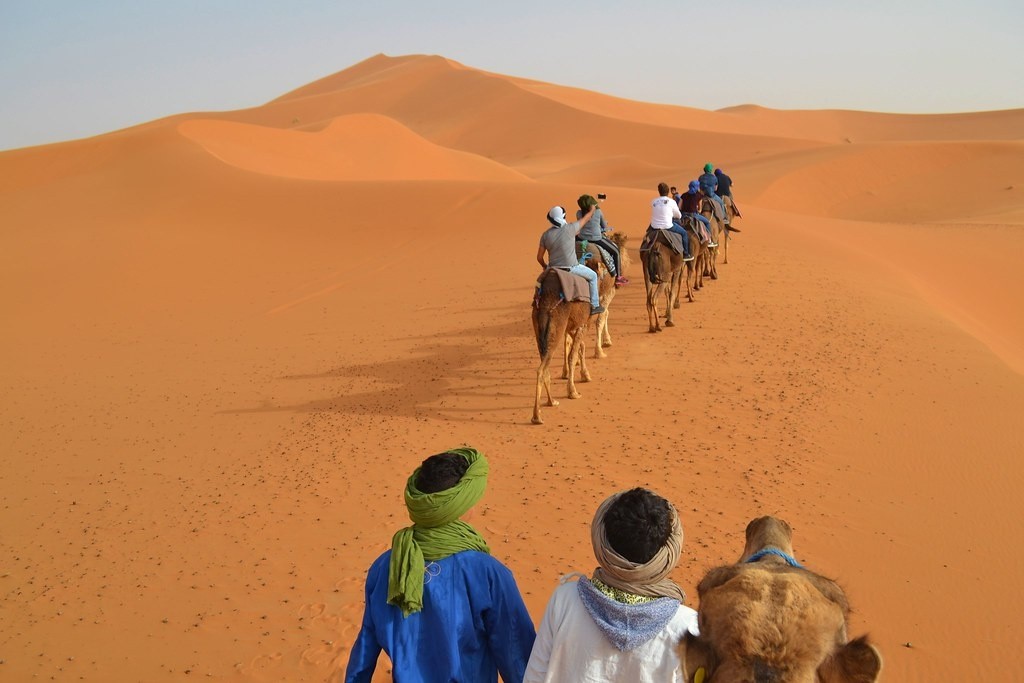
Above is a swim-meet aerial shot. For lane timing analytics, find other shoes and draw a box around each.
[614,276,629,285]
[708,242,719,247]
[683,255,695,262]
[590,306,606,315]
[723,218,730,225]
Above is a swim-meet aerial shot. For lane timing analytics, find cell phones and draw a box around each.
[597,193,607,200]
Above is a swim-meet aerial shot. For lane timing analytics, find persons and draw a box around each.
[537,204,605,314]
[650,163,733,261]
[575,195,629,285]
[523,487,701,683]
[344,448,537,683]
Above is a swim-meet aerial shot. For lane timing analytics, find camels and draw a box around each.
[675,514,885,683]
[639,191,740,333]
[531,230,633,425]
